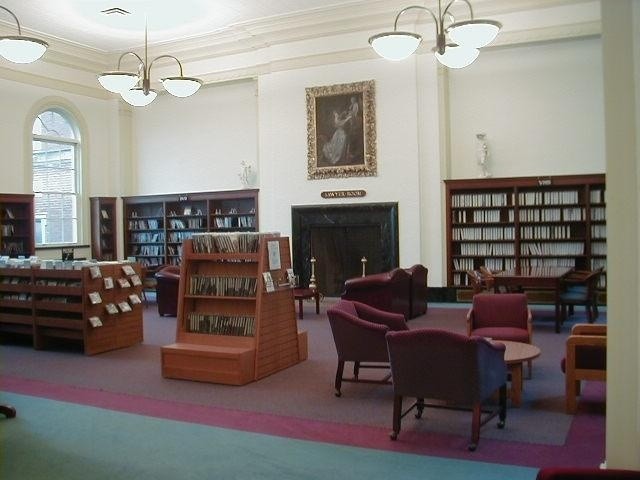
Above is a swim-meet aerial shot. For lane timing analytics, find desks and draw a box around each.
[485,338,542,408]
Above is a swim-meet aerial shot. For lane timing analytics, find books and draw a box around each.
[451,189,606,288]
[0,206,281,337]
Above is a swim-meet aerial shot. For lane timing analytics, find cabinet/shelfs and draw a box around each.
[1,193,35,258]
[1,258,145,356]
[161,232,308,386]
[90,197,117,259]
[441,173,608,334]
[120,189,259,290]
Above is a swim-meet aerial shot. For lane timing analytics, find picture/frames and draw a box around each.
[306,78,378,180]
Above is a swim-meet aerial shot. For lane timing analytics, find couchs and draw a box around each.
[153,266,181,317]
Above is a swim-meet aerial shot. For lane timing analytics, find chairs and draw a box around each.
[466,294,534,390]
[560,323,607,415]
[385,330,510,453]
[327,300,411,400]
[340,263,429,325]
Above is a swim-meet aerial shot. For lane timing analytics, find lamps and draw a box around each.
[366,0,503,70]
[0,6,48,65]
[97,10,203,108]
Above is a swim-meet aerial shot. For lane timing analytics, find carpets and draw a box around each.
[0,294,607,469]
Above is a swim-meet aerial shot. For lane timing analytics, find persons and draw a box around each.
[348,96,359,119]
[331,110,353,162]
